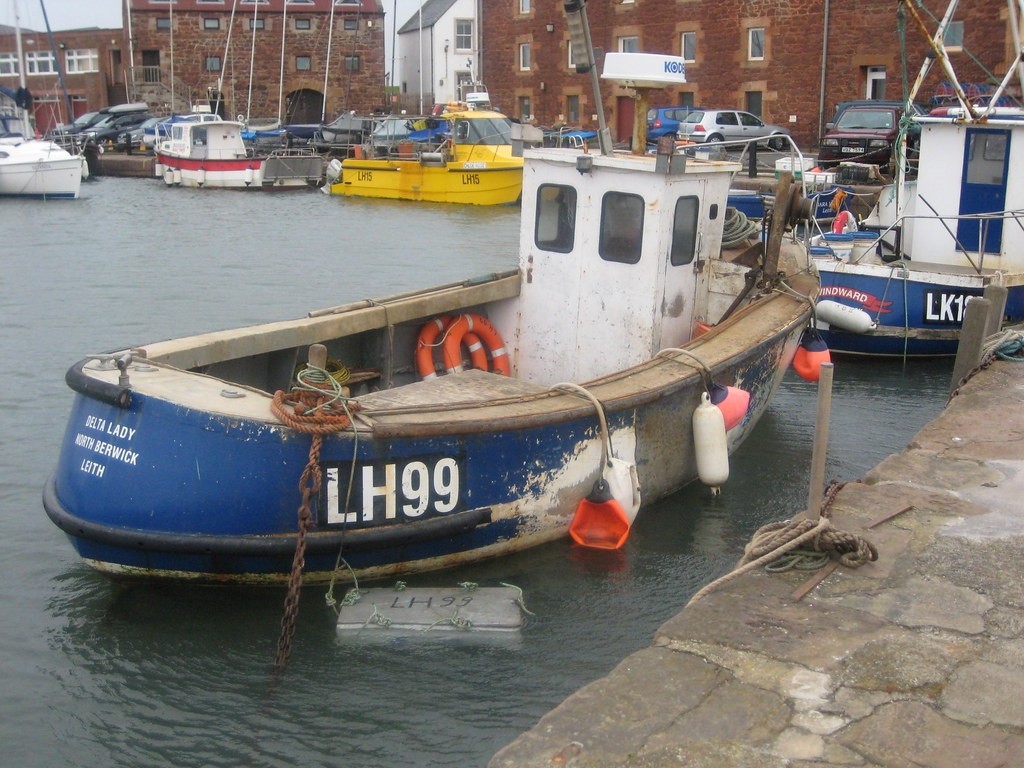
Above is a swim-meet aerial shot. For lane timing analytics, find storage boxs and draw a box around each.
[838,164,869,180]
[775,156,814,171]
[804,171,836,183]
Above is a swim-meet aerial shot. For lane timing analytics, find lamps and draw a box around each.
[546,23,554,32]
[367,19,373,29]
[540,80,546,91]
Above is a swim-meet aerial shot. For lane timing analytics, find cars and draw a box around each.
[818,106,914,174]
[824,100,931,154]
[51,112,108,143]
[645,106,706,144]
[117,117,167,147]
[676,110,794,151]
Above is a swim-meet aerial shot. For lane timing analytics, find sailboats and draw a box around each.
[0,0,91,200]
[141,1,255,149]
[367,0,431,151]
[254,0,317,147]
[309,0,380,142]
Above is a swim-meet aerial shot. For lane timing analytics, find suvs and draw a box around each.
[76,103,159,152]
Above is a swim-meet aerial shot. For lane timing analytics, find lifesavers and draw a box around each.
[442,312,511,378]
[414,315,490,382]
[830,210,859,236]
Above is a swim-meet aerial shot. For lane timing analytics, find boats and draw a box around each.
[806,1,1024,357]
[154,120,324,191]
[676,135,856,224]
[321,109,543,204]
[42,2,832,586]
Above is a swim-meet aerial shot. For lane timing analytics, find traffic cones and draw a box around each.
[108,139,115,152]
